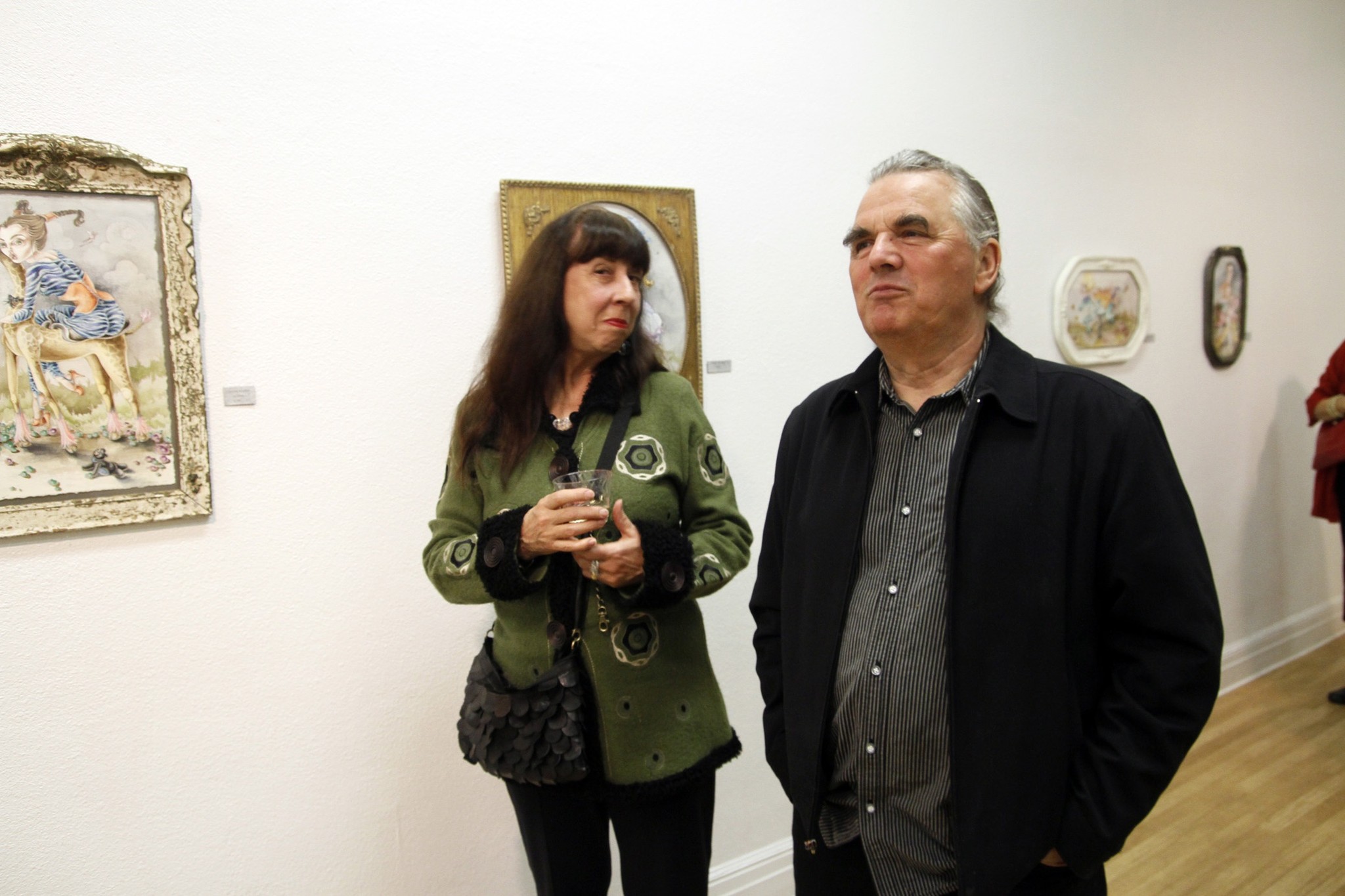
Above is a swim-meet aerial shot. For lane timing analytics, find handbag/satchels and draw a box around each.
[456,644,602,798]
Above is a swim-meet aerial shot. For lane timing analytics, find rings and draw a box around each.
[591,572,598,580]
[588,560,600,575]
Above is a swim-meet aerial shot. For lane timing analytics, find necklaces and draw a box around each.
[535,410,607,481]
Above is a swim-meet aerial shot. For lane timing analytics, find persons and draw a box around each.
[747,148,1226,896]
[422,204,753,895]
[1304,337,1345,705]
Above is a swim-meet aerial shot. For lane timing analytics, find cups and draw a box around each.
[553,469,611,523]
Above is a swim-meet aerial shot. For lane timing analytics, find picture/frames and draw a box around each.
[499,179,704,409]
[0,132,214,540]
[1202,246,1247,369]
[1052,252,1155,367]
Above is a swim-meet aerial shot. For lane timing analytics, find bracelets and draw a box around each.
[1325,393,1345,420]
